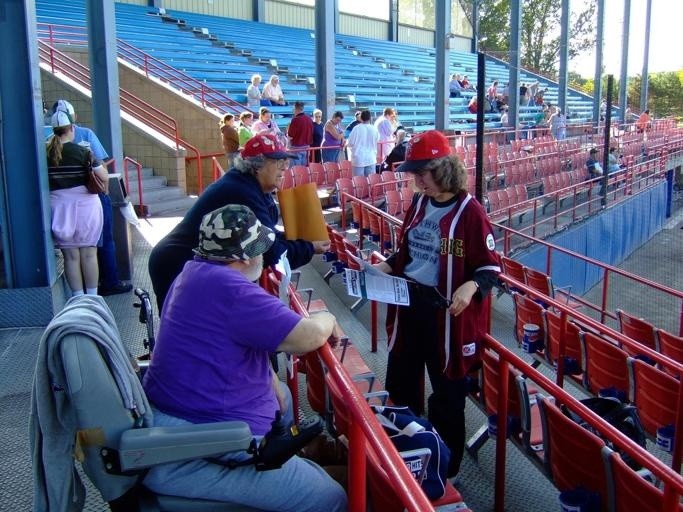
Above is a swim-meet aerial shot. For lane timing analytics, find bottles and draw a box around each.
[276,132,287,147]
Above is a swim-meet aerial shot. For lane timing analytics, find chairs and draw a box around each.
[260,112,682,511]
[50,326,252,512]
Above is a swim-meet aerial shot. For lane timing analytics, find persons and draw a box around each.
[247,73,272,107]
[624,103,632,132]
[44,110,106,296]
[373,128,504,487]
[449,73,566,139]
[587,148,613,195]
[636,110,650,133]
[140,203,349,512]
[45,99,134,297]
[599,98,606,122]
[218,104,410,179]
[261,75,292,118]
[147,135,332,321]
[609,146,622,187]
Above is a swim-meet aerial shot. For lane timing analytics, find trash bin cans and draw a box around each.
[446,129,465,147]
[97,173,133,280]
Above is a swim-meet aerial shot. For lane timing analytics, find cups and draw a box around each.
[78,141,90,151]
[522,321,540,342]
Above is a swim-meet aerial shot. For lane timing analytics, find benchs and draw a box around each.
[479,50,640,140]
[33,1,478,166]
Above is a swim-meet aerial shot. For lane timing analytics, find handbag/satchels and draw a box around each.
[369,402,455,503]
[87,164,107,195]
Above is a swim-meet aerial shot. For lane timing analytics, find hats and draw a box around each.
[51,109,74,128]
[191,203,277,263]
[52,98,75,115]
[393,128,452,174]
[590,149,600,154]
[239,132,301,162]
